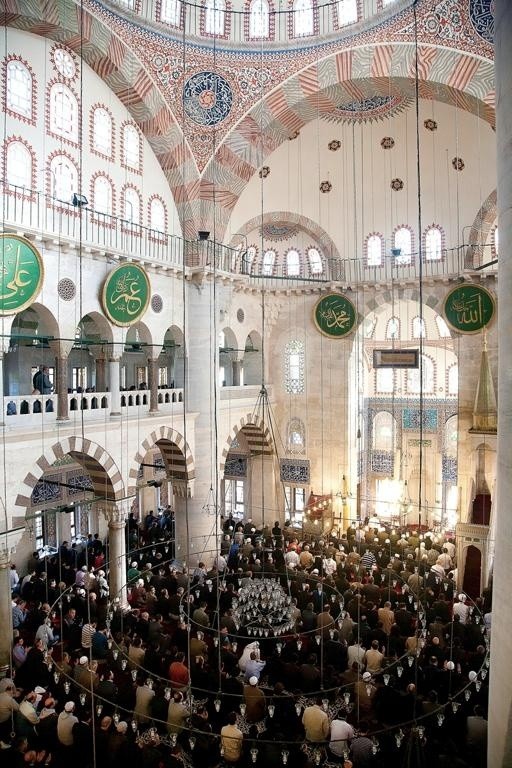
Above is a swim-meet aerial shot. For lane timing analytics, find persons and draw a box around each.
[33,363,183,412]
[1,503,489,767]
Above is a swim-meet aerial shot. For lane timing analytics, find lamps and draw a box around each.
[73,193,88,206]
[199,231,210,240]
[36,0,489,764]
[391,249,400,256]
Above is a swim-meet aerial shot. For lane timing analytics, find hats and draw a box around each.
[250,676,258,685]
[225,535,230,540]
[458,594,467,602]
[395,554,400,558]
[174,692,183,699]
[469,670,477,682]
[420,542,428,560]
[82,566,105,578]
[136,579,144,587]
[374,534,407,544]
[255,559,261,564]
[229,526,234,530]
[79,656,88,664]
[34,686,45,694]
[319,541,324,546]
[246,519,255,543]
[447,661,454,670]
[118,721,127,733]
[65,701,75,712]
[363,672,371,681]
[408,684,417,693]
[132,562,137,567]
[304,545,309,550]
[246,571,252,577]
[177,587,184,594]
